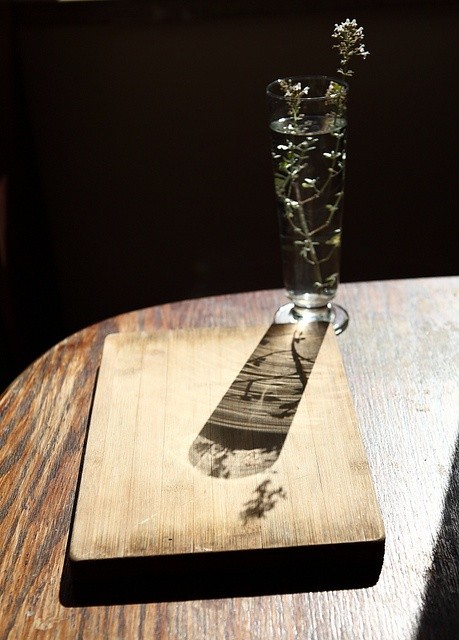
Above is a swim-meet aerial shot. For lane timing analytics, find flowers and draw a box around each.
[272,18,371,299]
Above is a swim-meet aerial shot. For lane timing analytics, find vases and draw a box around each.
[265,75,351,336]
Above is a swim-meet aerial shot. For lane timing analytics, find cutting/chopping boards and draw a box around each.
[68,320,388,565]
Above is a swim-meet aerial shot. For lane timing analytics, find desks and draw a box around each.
[1,274,459,640]
